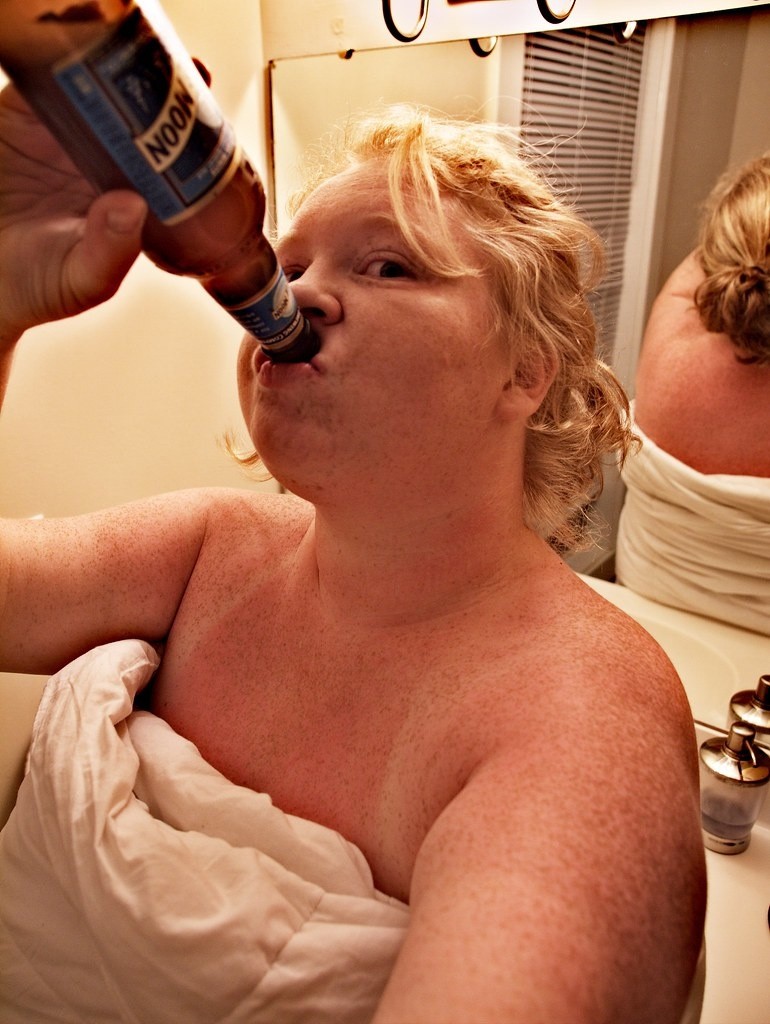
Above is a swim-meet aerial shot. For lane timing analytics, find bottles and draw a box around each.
[0,1,318,365]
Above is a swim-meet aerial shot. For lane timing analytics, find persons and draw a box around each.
[619,154,770,636]
[0,58,706,1023]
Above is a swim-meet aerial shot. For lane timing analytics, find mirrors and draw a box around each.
[267,0,770,752]
